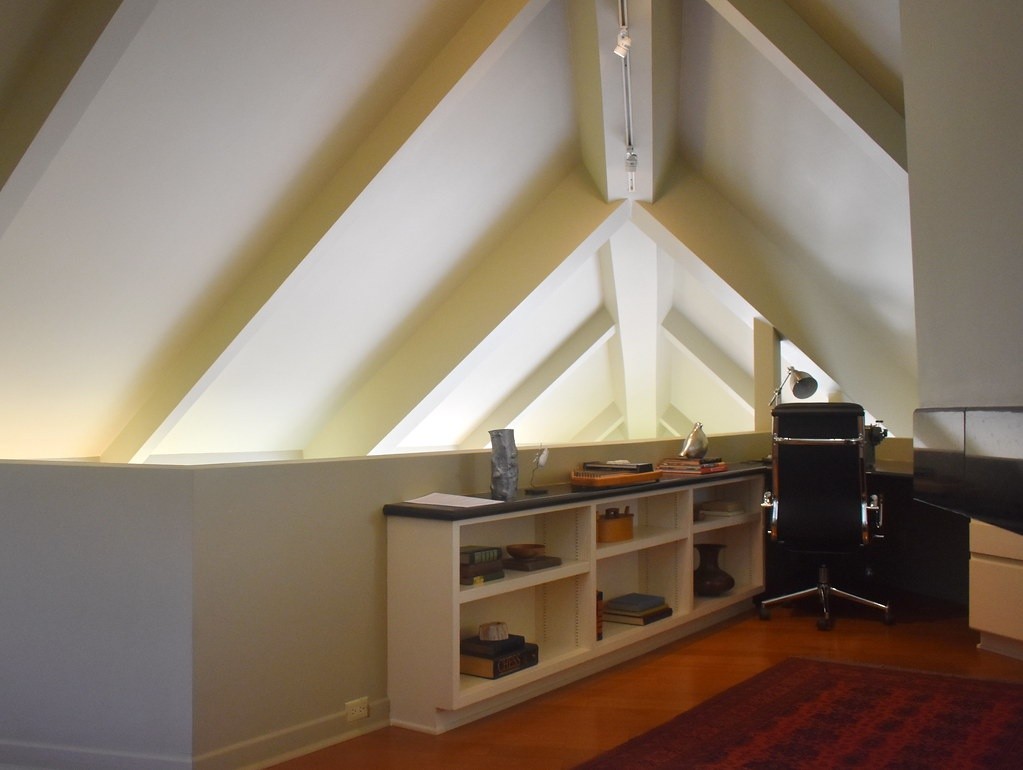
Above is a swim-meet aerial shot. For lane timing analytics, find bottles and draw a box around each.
[694,543,735,596]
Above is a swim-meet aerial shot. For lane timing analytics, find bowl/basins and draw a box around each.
[506,544,546,561]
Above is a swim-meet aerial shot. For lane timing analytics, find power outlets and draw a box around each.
[346,697,368,722]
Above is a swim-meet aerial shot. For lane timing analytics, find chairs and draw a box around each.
[757,399,894,630]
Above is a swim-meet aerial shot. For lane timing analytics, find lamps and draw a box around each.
[769,366,818,459]
[613,28,632,58]
[625,153,638,172]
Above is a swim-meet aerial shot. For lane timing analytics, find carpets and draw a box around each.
[578,656,1023,770]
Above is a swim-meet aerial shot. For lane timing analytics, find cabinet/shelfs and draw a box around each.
[384,462,771,736]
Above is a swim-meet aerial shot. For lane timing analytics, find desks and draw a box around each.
[878,452,1023,661]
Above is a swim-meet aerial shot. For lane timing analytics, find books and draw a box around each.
[657,457,728,474]
[460,633,539,679]
[597,591,603,641]
[603,593,672,625]
[459,546,561,585]
